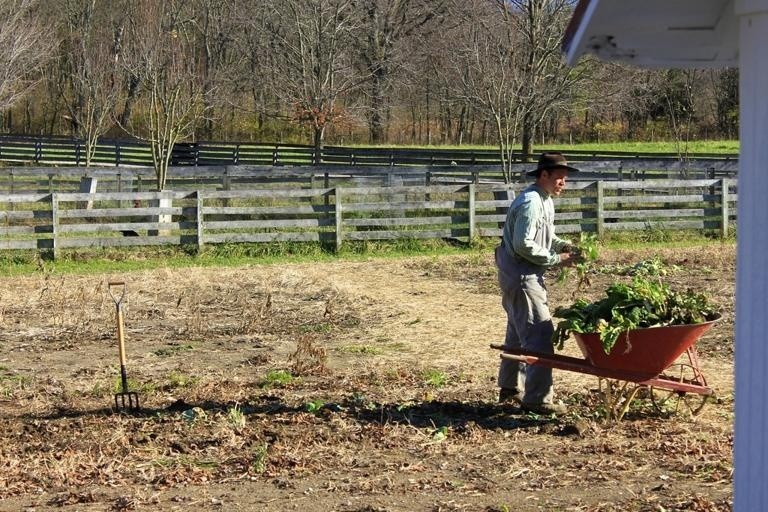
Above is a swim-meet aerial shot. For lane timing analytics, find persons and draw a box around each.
[495,151,583,416]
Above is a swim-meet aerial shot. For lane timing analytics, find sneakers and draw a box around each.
[520,401,567,415]
[499,387,523,404]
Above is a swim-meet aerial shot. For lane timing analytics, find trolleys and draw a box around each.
[491,294,728,421]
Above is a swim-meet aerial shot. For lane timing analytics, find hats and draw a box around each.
[526,152,579,175]
